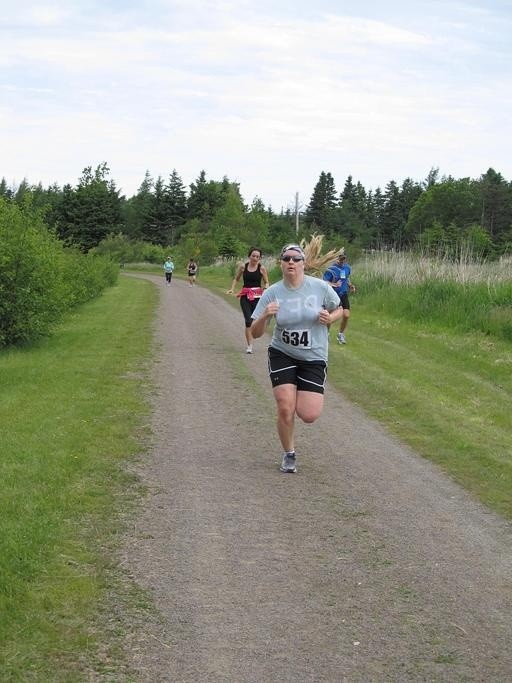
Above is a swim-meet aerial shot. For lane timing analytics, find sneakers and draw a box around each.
[336,332,346,346]
[246,343,254,354]
[165,281,170,287]
[278,448,297,472]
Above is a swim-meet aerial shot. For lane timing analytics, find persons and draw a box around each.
[163,257,176,288]
[250,232,345,473]
[225,247,270,355]
[186,257,199,288]
[322,256,356,345]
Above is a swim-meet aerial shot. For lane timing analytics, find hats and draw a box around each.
[336,254,346,260]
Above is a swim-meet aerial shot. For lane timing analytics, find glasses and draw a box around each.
[279,255,304,261]
